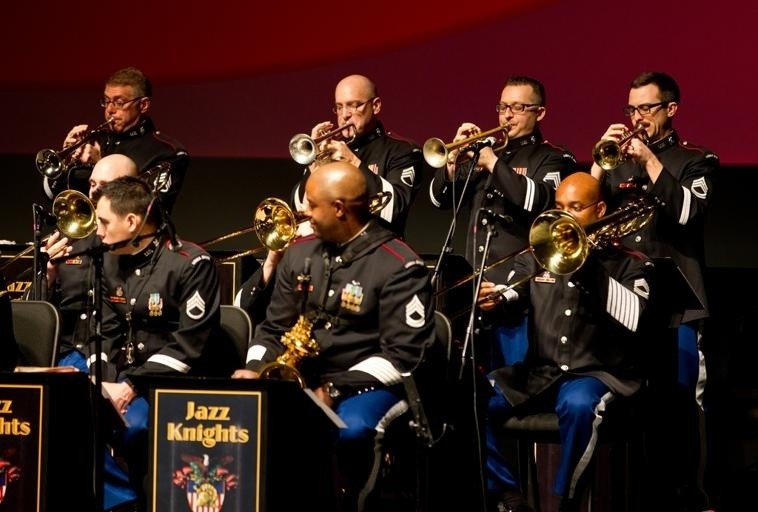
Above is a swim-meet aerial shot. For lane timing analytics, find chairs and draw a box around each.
[11,301,60,367]
[219,305,252,353]
[432,310,452,363]
[504,406,635,512]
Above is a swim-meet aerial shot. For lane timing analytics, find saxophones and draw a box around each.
[257,312,321,388]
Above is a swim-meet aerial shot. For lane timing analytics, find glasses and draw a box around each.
[101,96,143,109]
[623,103,662,116]
[496,102,541,114]
[333,98,374,114]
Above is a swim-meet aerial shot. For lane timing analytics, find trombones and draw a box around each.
[0,161,172,292]
[434,195,664,320]
[198,191,392,264]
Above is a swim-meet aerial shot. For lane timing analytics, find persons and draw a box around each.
[475,172,654,512]
[232,250,282,309]
[289,74,423,236]
[58,176,220,512]
[590,70,719,512]
[429,75,577,492]
[63,66,189,216]
[23,154,137,374]
[231,162,435,512]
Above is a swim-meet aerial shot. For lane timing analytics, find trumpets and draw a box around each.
[289,121,357,165]
[35,118,116,180]
[423,122,511,169]
[592,123,651,170]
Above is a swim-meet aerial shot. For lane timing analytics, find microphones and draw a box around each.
[482,206,514,224]
[459,136,498,155]
[160,204,184,253]
[140,149,190,178]
